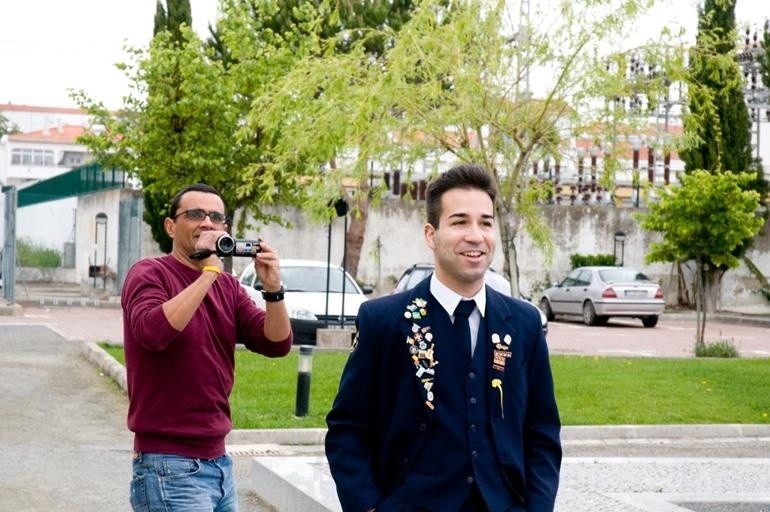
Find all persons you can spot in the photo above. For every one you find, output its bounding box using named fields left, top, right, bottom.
left=325, top=166, right=565, bottom=512
left=118, top=184, right=295, bottom=511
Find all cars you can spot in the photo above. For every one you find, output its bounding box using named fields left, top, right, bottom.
left=536, top=265, right=667, bottom=328
left=391, top=261, right=549, bottom=340
left=238, top=258, right=374, bottom=345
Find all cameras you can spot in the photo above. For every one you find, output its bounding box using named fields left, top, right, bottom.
left=215, top=234, right=261, bottom=258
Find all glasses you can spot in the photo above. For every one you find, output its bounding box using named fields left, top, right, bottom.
left=174, top=209, right=225, bottom=225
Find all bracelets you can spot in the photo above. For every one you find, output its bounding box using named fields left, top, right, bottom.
left=200, top=265, right=224, bottom=276
left=259, top=284, right=286, bottom=302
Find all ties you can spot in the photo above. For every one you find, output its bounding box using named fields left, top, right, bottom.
left=454, top=301, right=478, bottom=358
